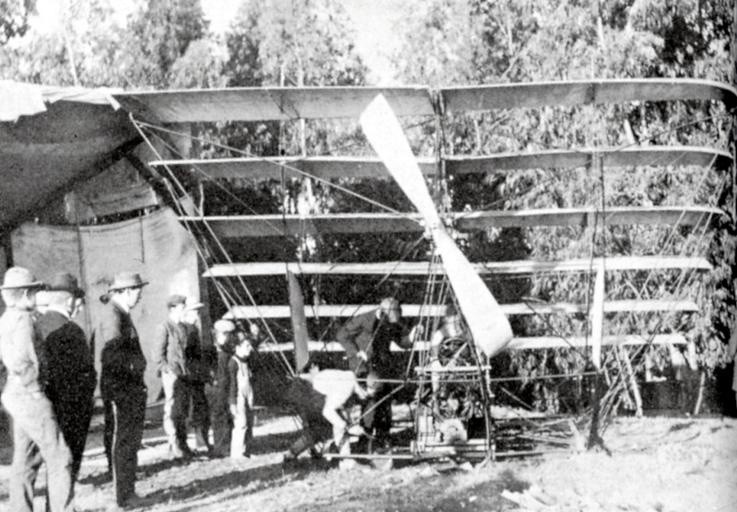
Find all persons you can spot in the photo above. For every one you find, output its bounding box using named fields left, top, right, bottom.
left=154, top=294, right=257, bottom=462
left=283, top=298, right=426, bottom=467
left=0, top=267, right=150, bottom=512
left=726, top=277, right=737, bottom=408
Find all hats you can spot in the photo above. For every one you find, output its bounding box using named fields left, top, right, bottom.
left=167, top=295, right=186, bottom=306
left=214, top=320, right=235, bottom=333
left=0, top=266, right=43, bottom=289
left=46, top=272, right=85, bottom=297
left=109, top=272, right=148, bottom=292
